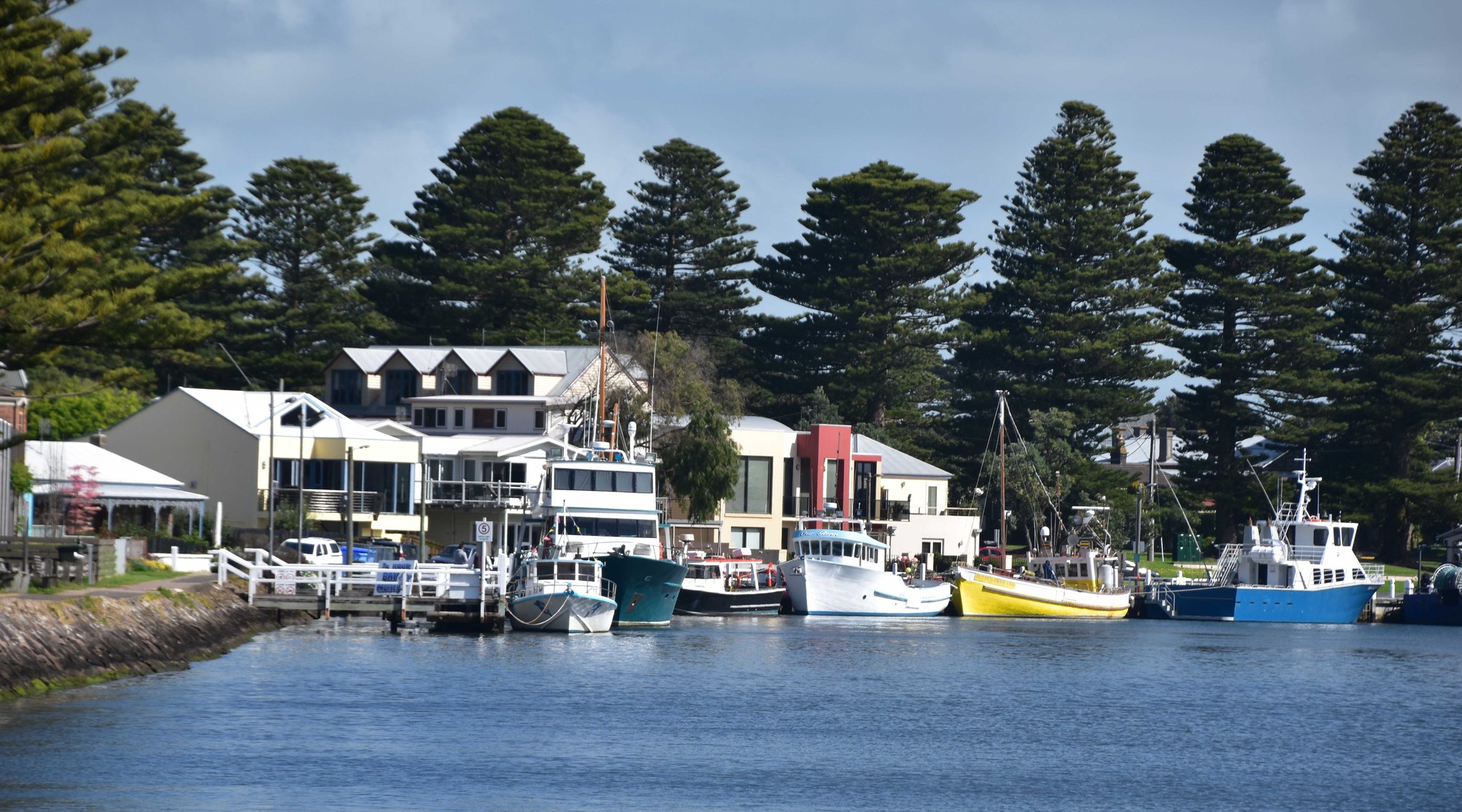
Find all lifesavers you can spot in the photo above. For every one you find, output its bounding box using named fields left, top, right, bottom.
left=739, top=572, right=750, bottom=583
left=725, top=573, right=741, bottom=589
left=1307, top=518, right=1321, bottom=522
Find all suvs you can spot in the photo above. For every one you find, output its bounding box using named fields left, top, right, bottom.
left=373, top=538, right=428, bottom=564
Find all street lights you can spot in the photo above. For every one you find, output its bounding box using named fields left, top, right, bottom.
left=345, top=443, right=369, bottom=588
left=267, top=391, right=298, bottom=591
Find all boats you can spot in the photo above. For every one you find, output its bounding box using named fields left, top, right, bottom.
left=530, top=275, right=690, bottom=627
left=1142, top=447, right=1385, bottom=625
left=673, top=533, right=786, bottom=618
left=507, top=498, right=617, bottom=633
left=776, top=432, right=955, bottom=617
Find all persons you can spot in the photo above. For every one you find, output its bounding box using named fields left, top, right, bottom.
left=453, top=543, right=468, bottom=564
left=531, top=546, right=539, bottom=559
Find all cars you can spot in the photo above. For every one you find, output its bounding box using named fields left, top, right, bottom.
left=979, top=548, right=1003, bottom=562
left=432, top=542, right=477, bottom=567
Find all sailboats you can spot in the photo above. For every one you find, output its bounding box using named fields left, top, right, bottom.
left=951, top=391, right=1134, bottom=620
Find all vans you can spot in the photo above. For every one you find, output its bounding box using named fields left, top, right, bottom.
left=276, top=537, right=343, bottom=573
